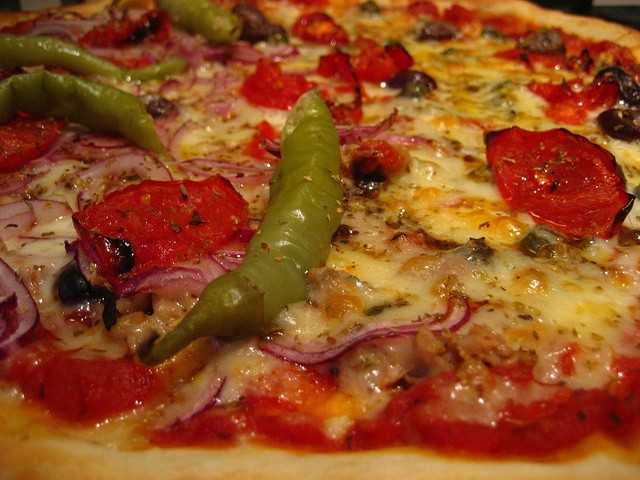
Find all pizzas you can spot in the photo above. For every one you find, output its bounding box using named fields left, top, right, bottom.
left=0, top=0, right=640, bottom=479
